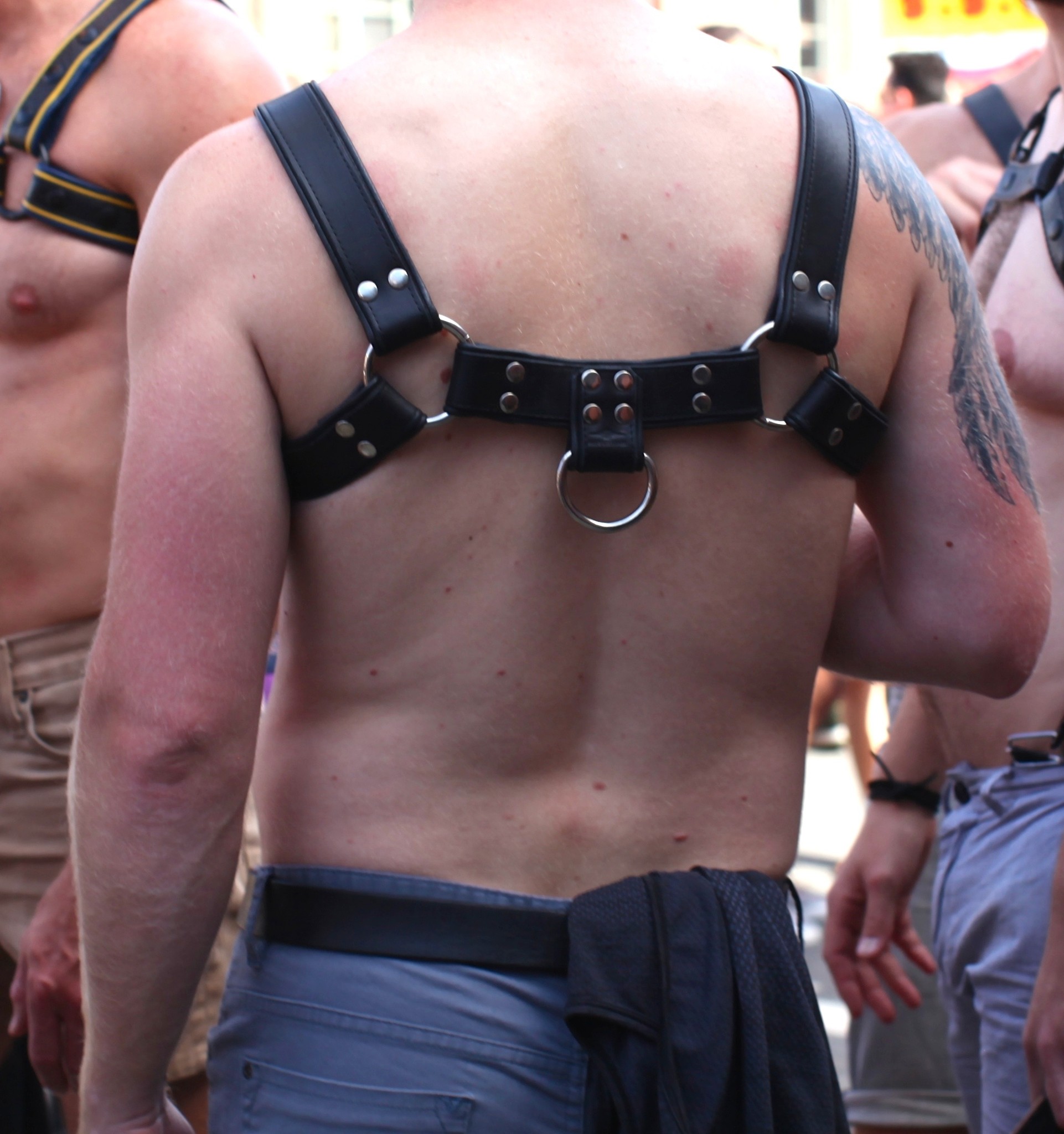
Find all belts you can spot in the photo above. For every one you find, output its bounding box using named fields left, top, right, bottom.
left=238, top=869, right=567, bottom=970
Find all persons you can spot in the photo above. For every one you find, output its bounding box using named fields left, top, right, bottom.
left=0, top=0, right=1064, bottom=1134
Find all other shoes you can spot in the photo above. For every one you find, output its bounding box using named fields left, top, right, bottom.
left=811, top=696, right=851, bottom=747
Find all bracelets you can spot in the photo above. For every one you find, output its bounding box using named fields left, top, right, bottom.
left=863, top=748, right=946, bottom=814
